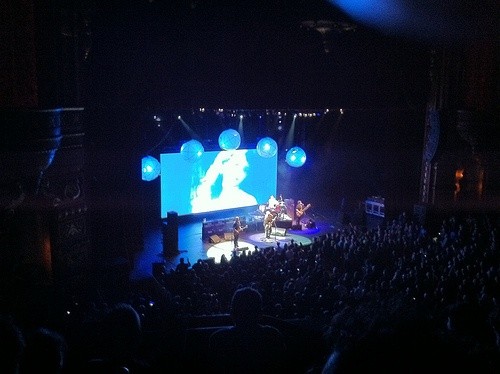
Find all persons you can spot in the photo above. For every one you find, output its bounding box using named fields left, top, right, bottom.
left=191, top=150, right=259, bottom=214
left=0, top=196, right=500, bottom=374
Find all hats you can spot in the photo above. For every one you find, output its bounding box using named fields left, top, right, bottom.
left=231, top=287, right=263, bottom=324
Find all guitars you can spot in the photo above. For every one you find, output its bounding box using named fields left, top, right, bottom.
left=296, top=203, right=311, bottom=217
left=233, top=225, right=248, bottom=236
left=266, top=217, right=276, bottom=230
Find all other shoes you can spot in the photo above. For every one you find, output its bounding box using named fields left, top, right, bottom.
left=266, top=236, right=270, bottom=238
